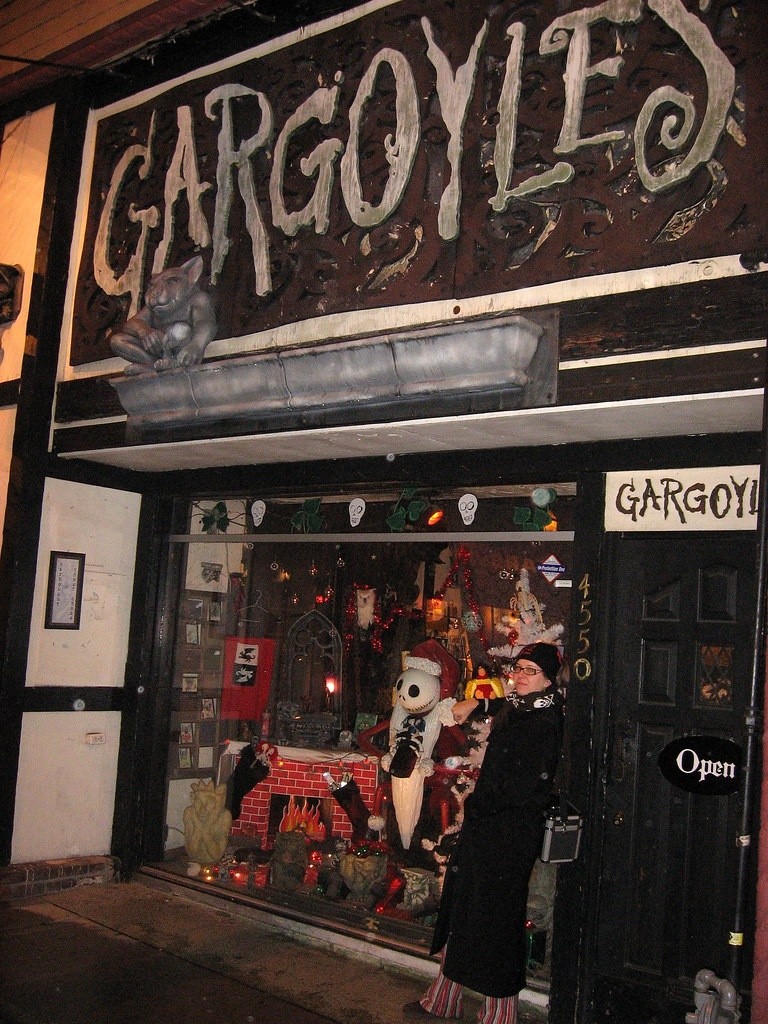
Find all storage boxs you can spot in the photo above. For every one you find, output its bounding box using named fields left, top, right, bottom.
left=536, top=795, right=584, bottom=863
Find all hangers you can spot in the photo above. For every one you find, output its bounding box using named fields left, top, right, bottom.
left=238, top=590, right=285, bottom=624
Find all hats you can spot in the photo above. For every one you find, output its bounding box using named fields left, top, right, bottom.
left=515, top=643, right=561, bottom=684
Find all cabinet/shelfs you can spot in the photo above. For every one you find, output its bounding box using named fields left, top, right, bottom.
left=167, top=589, right=229, bottom=780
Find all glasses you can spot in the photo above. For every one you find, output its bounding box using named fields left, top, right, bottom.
left=510, top=662, right=543, bottom=675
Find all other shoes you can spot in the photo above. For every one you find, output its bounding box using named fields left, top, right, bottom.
left=402, top=1000, right=435, bottom=1020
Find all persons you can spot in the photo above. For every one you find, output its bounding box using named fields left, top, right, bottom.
left=403, top=644, right=567, bottom=1024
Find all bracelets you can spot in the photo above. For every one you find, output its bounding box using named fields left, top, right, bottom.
left=484, top=698, right=489, bottom=713
